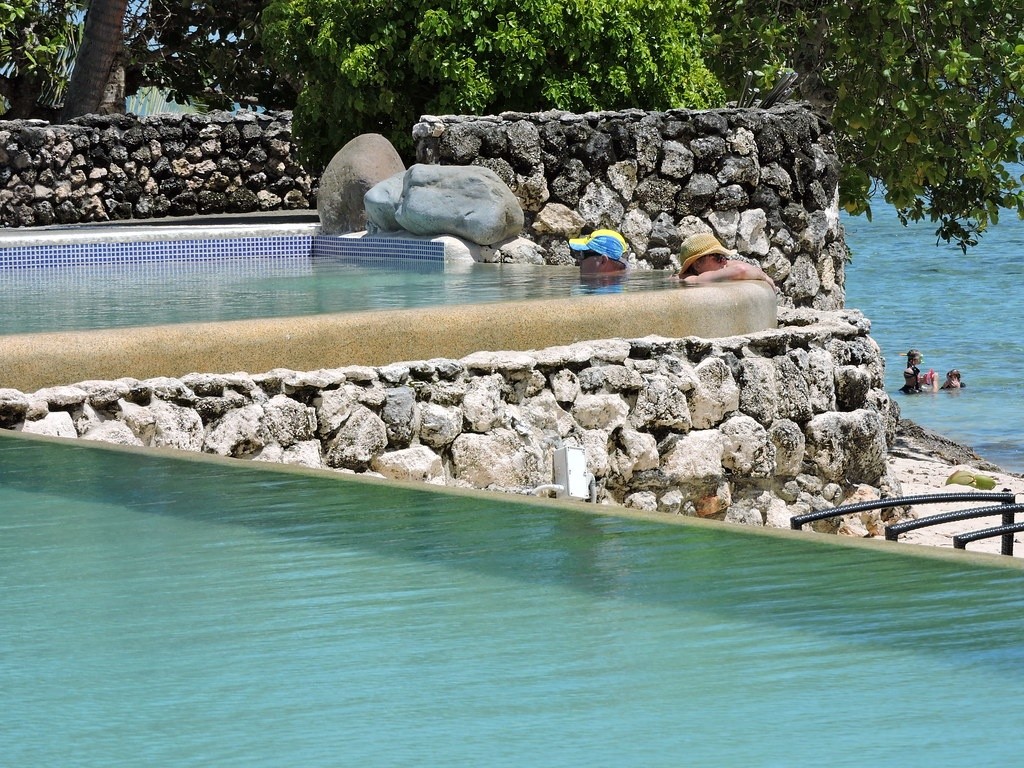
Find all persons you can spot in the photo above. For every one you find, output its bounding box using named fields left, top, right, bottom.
left=569, top=229, right=629, bottom=273
left=674, top=233, right=777, bottom=294
left=899, top=349, right=967, bottom=395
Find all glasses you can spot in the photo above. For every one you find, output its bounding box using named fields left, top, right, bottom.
left=580, top=250, right=602, bottom=260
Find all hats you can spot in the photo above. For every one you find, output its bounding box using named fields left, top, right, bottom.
left=679, top=233, right=731, bottom=279
left=568, top=230, right=626, bottom=261
